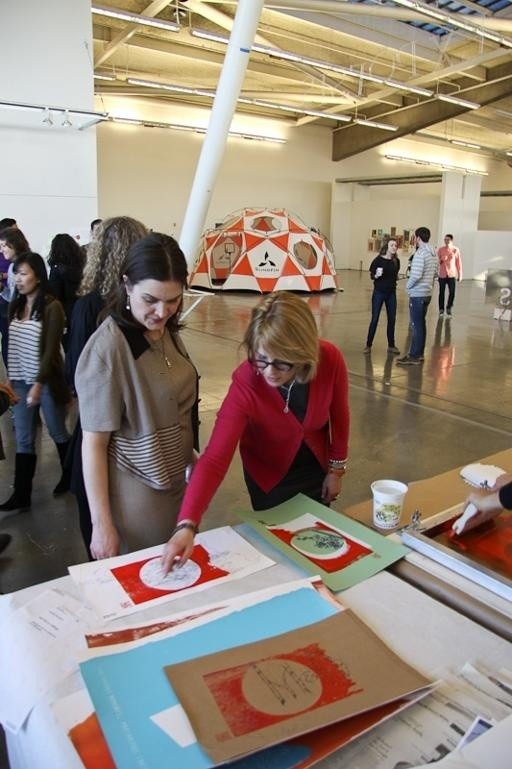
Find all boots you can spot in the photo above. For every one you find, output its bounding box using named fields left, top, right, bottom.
left=1, top=453, right=37, bottom=512
left=53, top=436, right=72, bottom=496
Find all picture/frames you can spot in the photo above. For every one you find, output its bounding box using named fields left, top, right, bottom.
left=399, top=500, right=512, bottom=603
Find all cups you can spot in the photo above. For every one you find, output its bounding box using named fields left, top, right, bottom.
left=376, top=268, right=383, bottom=277
left=370, top=479, right=408, bottom=530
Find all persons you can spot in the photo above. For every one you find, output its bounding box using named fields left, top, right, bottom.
left=466, top=473, right=511, bottom=517
left=361, top=239, right=401, bottom=353
left=0, top=218, right=147, bottom=563
left=407, top=243, right=419, bottom=277
left=75, top=233, right=201, bottom=560
left=436, top=235, right=462, bottom=316
left=160, top=290, right=349, bottom=579
left=396, top=227, right=439, bottom=365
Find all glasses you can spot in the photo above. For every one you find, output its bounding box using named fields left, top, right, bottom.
left=246, top=348, right=294, bottom=371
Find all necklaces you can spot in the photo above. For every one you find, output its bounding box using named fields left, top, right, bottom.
left=279, top=380, right=296, bottom=413
left=147, top=329, right=171, bottom=368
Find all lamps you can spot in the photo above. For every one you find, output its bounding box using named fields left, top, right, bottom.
left=41, top=103, right=75, bottom=131
left=384, top=117, right=512, bottom=177
left=93, top=0, right=512, bottom=144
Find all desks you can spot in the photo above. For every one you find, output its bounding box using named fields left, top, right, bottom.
left=328, top=446, right=512, bottom=642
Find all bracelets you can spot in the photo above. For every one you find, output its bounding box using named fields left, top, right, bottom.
left=170, top=524, right=196, bottom=539
left=329, top=466, right=347, bottom=475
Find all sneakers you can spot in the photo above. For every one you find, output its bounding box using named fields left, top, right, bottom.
left=439, top=310, right=452, bottom=318
left=365, top=345, right=424, bottom=365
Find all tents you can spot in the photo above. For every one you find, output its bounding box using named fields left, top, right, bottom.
left=187, top=207, right=343, bottom=293
